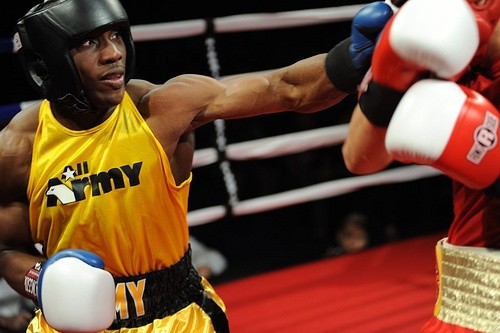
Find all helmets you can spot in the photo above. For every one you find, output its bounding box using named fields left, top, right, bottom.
left=12, top=0, right=134, bottom=118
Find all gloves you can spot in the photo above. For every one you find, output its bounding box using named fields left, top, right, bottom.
left=325, top=0, right=408, bottom=94
left=358, top=0, right=491, bottom=127
left=38, top=248, right=117, bottom=332
left=385, top=79, right=499, bottom=197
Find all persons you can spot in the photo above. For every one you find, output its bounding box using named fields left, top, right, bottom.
left=0, top=276, right=39, bottom=333
left=186, top=233, right=227, bottom=281
left=0, top=0, right=399, bottom=333
left=341, top=0, right=500, bottom=333
left=336, top=213, right=369, bottom=255
